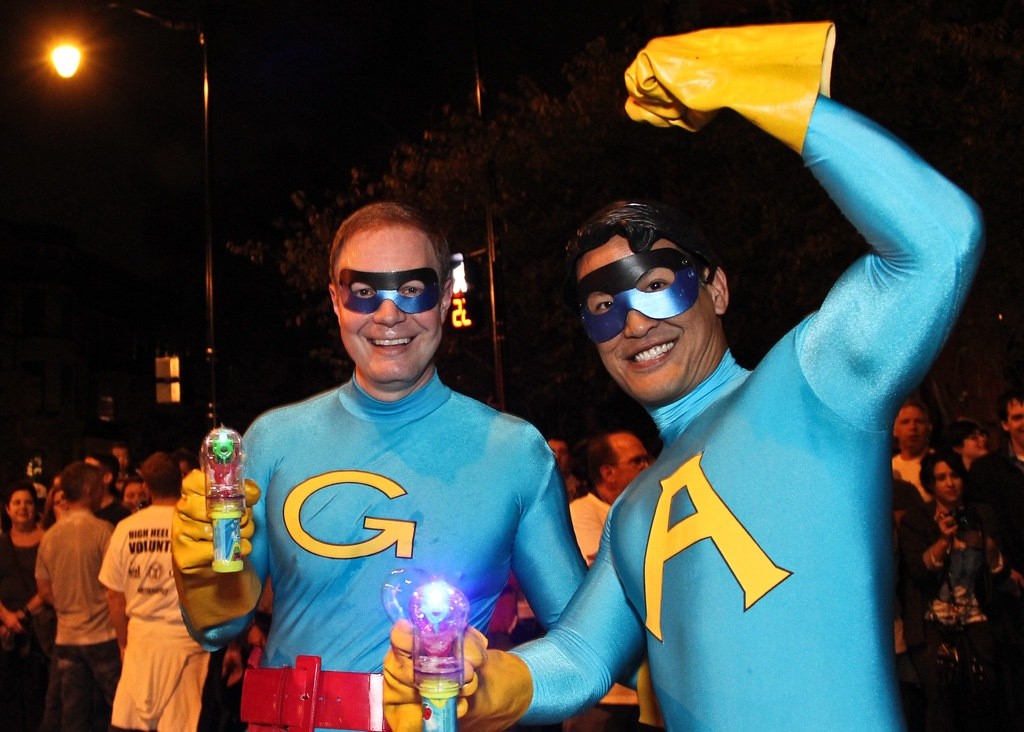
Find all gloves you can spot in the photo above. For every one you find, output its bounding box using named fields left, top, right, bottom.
left=385, top=618, right=534, bottom=732
left=623, top=20, right=836, bottom=154
left=171, top=466, right=262, bottom=631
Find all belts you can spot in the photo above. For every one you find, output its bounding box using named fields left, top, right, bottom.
left=238, top=654, right=394, bottom=732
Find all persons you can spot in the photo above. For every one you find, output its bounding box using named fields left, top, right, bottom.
left=968, top=385, right=1023, bottom=732
left=568, top=431, right=656, bottom=732
left=1, top=433, right=588, bottom=732
left=891, top=477, right=930, bottom=732
left=173, top=204, right=667, bottom=732
left=896, top=446, right=1011, bottom=732
left=889, top=394, right=945, bottom=503
left=944, top=422, right=991, bottom=472
left=380, top=19, right=984, bottom=732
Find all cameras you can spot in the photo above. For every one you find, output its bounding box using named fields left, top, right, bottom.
left=946, top=502, right=983, bottom=533
left=137, top=500, right=149, bottom=511
